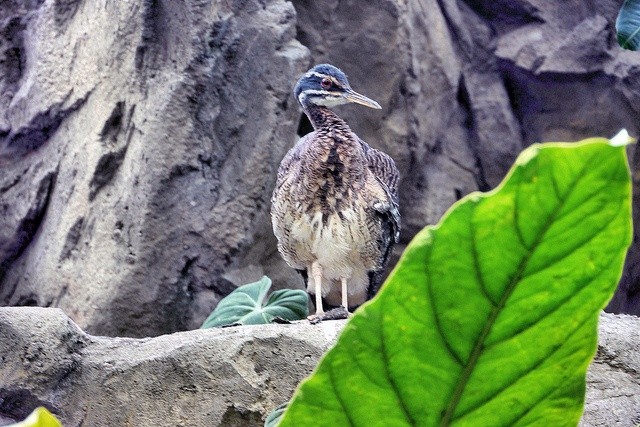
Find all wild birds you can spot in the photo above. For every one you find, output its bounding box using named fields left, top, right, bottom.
left=269, top=63, right=403, bottom=324
